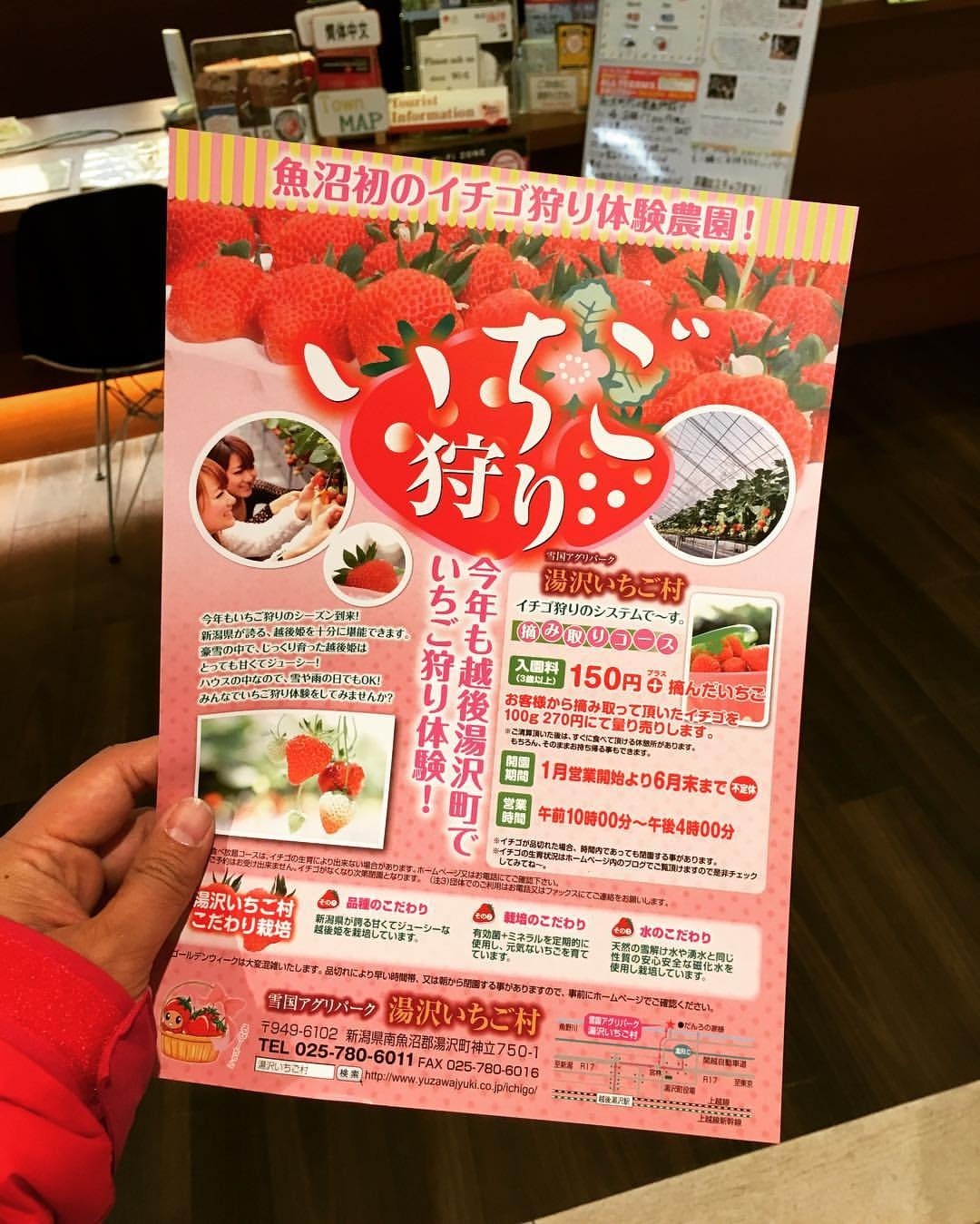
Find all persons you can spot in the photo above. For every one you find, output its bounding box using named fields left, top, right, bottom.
left=195, top=434, right=346, bottom=566
left=0, top=731, right=216, bottom=1224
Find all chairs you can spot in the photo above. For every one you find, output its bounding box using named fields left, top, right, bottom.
left=19, top=182, right=166, bottom=560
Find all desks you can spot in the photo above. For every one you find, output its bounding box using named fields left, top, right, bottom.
left=0, top=96, right=588, bottom=466
